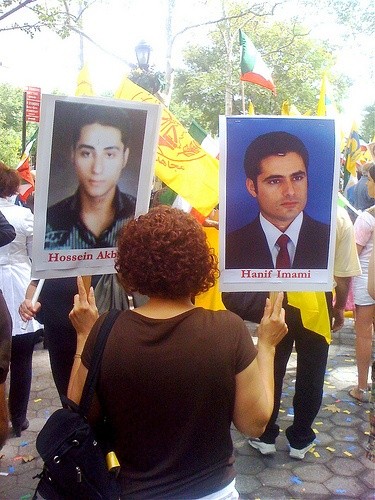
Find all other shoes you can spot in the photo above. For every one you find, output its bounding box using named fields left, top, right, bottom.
left=348, top=387, right=371, bottom=402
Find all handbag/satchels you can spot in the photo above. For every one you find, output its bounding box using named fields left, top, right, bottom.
left=32, top=395, right=124, bottom=500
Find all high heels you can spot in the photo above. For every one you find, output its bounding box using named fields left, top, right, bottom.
left=14, top=418, right=30, bottom=437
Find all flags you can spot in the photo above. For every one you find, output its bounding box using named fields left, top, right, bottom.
left=239, top=28, right=277, bottom=93
left=161, top=121, right=220, bottom=224
left=15, top=127, right=38, bottom=201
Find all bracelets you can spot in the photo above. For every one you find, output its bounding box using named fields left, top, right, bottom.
left=74, top=353, right=82, bottom=358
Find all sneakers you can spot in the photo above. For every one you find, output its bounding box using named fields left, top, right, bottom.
left=289, top=441, right=314, bottom=459
left=248, top=436, right=277, bottom=456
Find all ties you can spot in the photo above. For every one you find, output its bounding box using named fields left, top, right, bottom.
left=275, top=234, right=291, bottom=269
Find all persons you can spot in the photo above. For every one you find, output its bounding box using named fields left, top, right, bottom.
left=44, top=106, right=136, bottom=249
left=0, top=162, right=375, bottom=462
left=67, top=206, right=289, bottom=500
left=225, top=131, right=330, bottom=269
